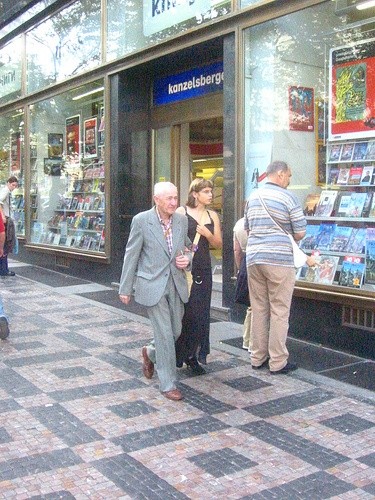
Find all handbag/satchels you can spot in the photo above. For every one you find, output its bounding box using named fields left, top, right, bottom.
left=291, top=236, right=310, bottom=270
left=185, top=269, right=192, bottom=298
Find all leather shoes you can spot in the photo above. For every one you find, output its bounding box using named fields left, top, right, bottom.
left=252, top=356, right=269, bottom=369
left=185, top=360, right=205, bottom=374
left=143, top=347, right=154, bottom=378
left=176, top=357, right=183, bottom=367
left=161, top=389, right=183, bottom=400
left=270, top=361, right=297, bottom=373
left=6, top=271, right=15, bottom=276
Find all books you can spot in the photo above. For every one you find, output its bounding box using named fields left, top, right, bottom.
left=11, top=155, right=105, bottom=253
left=296, top=140, right=375, bottom=289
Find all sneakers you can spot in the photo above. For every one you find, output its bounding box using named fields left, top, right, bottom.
left=0, top=316, right=9, bottom=339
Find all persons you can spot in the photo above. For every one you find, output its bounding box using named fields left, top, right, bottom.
left=233, top=201, right=321, bottom=353
left=244, top=161, right=307, bottom=374
left=85, top=129, right=95, bottom=155
left=176, top=178, right=222, bottom=375
left=0, top=176, right=18, bottom=275
left=0, top=209, right=10, bottom=340
left=67, top=132, right=76, bottom=155
left=119, top=182, right=192, bottom=401
